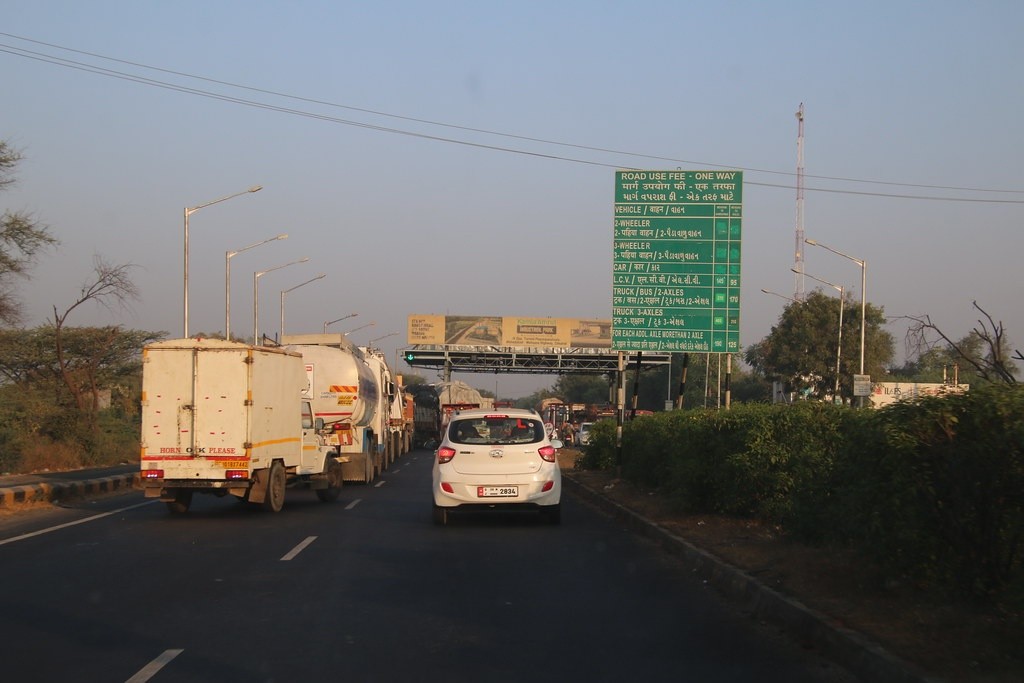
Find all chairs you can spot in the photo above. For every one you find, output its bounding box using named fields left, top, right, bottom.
left=458, top=427, right=482, bottom=442
left=508, top=422, right=530, bottom=437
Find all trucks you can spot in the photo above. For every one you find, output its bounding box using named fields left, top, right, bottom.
left=141, top=337, right=344, bottom=516
left=435, top=402, right=481, bottom=441
left=568, top=403, right=585, bottom=411
left=540, top=405, right=570, bottom=422
left=262, top=333, right=395, bottom=486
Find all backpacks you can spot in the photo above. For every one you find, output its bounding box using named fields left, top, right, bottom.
left=566, top=423, right=572, bottom=434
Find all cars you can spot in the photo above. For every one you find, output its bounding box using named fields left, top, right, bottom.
left=424, top=408, right=562, bottom=526
left=574, top=422, right=596, bottom=445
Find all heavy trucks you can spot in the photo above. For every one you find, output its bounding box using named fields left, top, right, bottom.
left=385, top=368, right=441, bottom=465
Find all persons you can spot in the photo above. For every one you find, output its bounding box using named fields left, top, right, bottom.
left=561, top=420, right=577, bottom=443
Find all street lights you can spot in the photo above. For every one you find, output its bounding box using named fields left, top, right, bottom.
left=369, top=331, right=400, bottom=348
left=792, top=268, right=844, bottom=405
left=805, top=239, right=866, bottom=408
left=183, top=185, right=266, bottom=337
left=281, top=273, right=327, bottom=335
left=225, top=232, right=289, bottom=341
left=254, top=259, right=310, bottom=346
left=343, top=322, right=376, bottom=336
left=761, top=288, right=802, bottom=402
left=323, top=314, right=358, bottom=334
